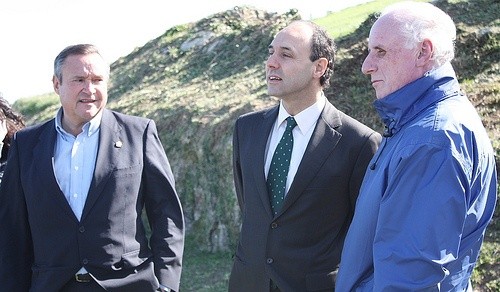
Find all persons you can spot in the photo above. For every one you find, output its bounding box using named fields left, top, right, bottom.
left=0, top=45, right=185, bottom=291
left=227, top=20, right=383, bottom=292
left=1, top=96, right=24, bottom=186
left=334, top=3, right=497, bottom=292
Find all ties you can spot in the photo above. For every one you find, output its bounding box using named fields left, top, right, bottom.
left=265, top=116, right=297, bottom=219
